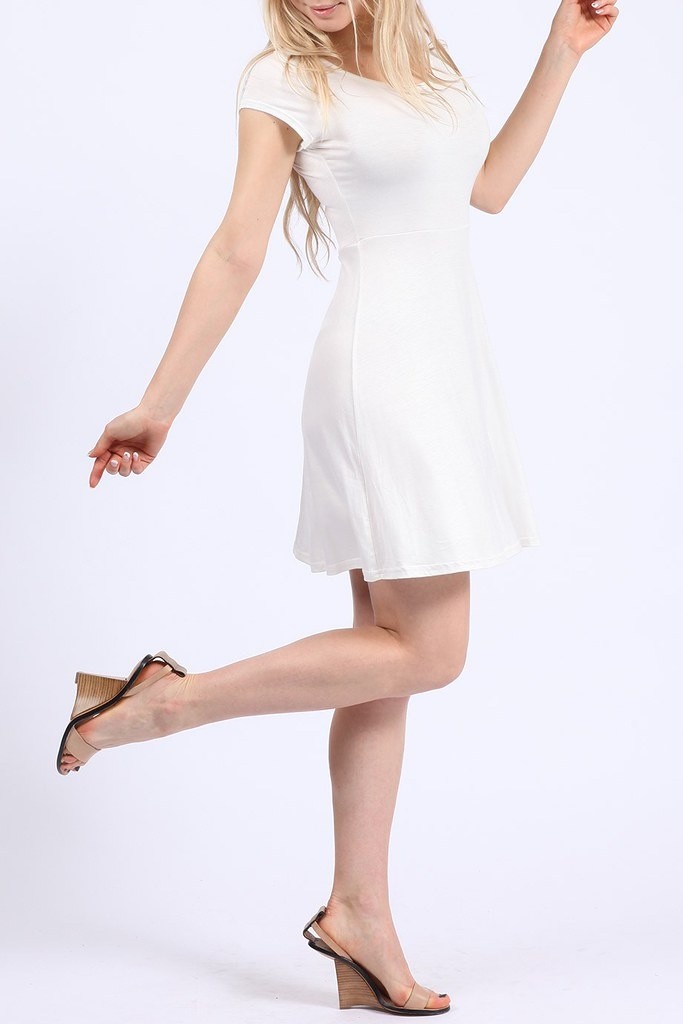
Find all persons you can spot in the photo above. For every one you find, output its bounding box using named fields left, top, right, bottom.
left=57, top=0, right=618, bottom=1016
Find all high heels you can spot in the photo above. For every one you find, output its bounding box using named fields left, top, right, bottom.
left=55, top=650, right=186, bottom=777
left=304, top=908, right=450, bottom=1016
